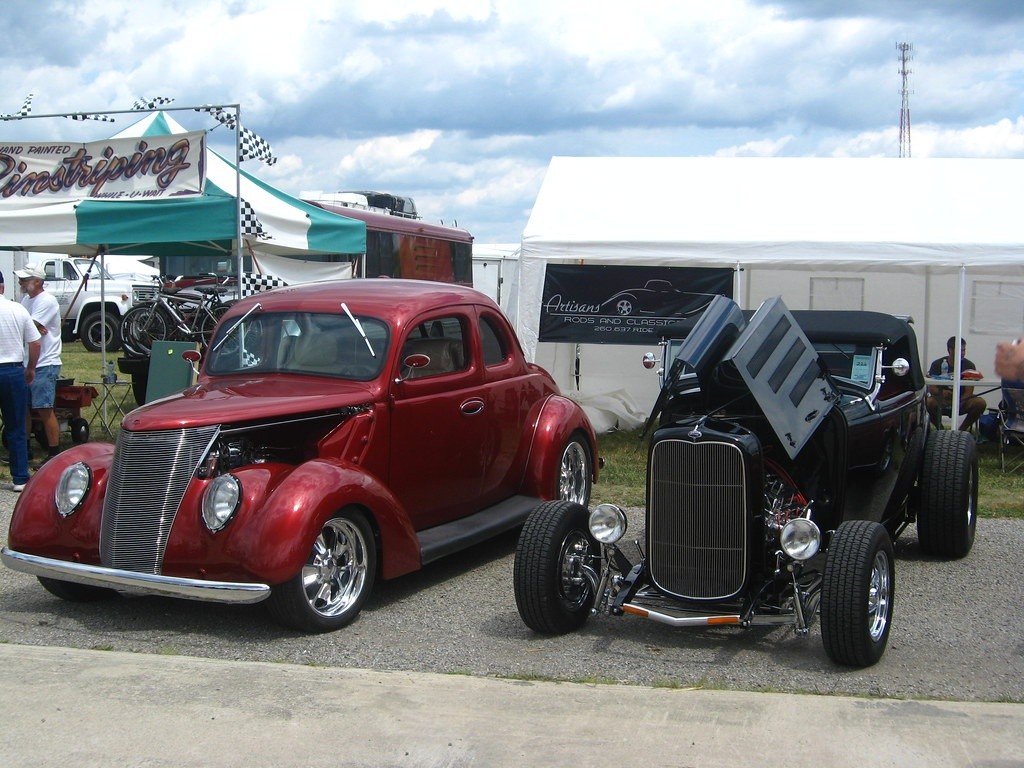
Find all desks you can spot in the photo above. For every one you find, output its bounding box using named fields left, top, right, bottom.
left=923, top=378, right=1002, bottom=445
left=79, top=381, right=136, bottom=439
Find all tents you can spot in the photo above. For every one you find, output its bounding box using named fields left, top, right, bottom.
left=0, top=109, right=368, bottom=426
left=472, top=154, right=1024, bottom=434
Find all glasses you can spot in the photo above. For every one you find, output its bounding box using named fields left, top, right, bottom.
left=19, top=277, right=35, bottom=281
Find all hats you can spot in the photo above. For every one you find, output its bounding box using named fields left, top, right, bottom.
left=13, top=263, right=46, bottom=280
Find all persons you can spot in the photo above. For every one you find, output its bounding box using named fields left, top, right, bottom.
left=994, top=338, right=1024, bottom=382
left=0, top=272, right=41, bottom=492
left=12, top=263, right=62, bottom=472
left=925, top=335, right=987, bottom=431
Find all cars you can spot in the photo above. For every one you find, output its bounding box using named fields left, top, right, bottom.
left=513, top=294, right=982, bottom=664
left=1, top=275, right=603, bottom=638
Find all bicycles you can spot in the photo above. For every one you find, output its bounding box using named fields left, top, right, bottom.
left=118, top=269, right=263, bottom=362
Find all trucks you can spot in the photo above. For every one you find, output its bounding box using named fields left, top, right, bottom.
left=1, top=250, right=160, bottom=352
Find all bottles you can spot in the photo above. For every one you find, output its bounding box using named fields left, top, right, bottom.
left=108, top=360, right=114, bottom=384
left=941, top=359, right=948, bottom=379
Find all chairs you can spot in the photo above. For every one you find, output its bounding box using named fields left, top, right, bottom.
left=919, top=392, right=978, bottom=443
left=1000, top=387, right=1024, bottom=472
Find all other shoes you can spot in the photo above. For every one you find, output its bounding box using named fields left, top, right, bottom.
left=33, top=455, right=54, bottom=471
left=1, top=449, right=33, bottom=463
left=13, top=483, right=25, bottom=492
